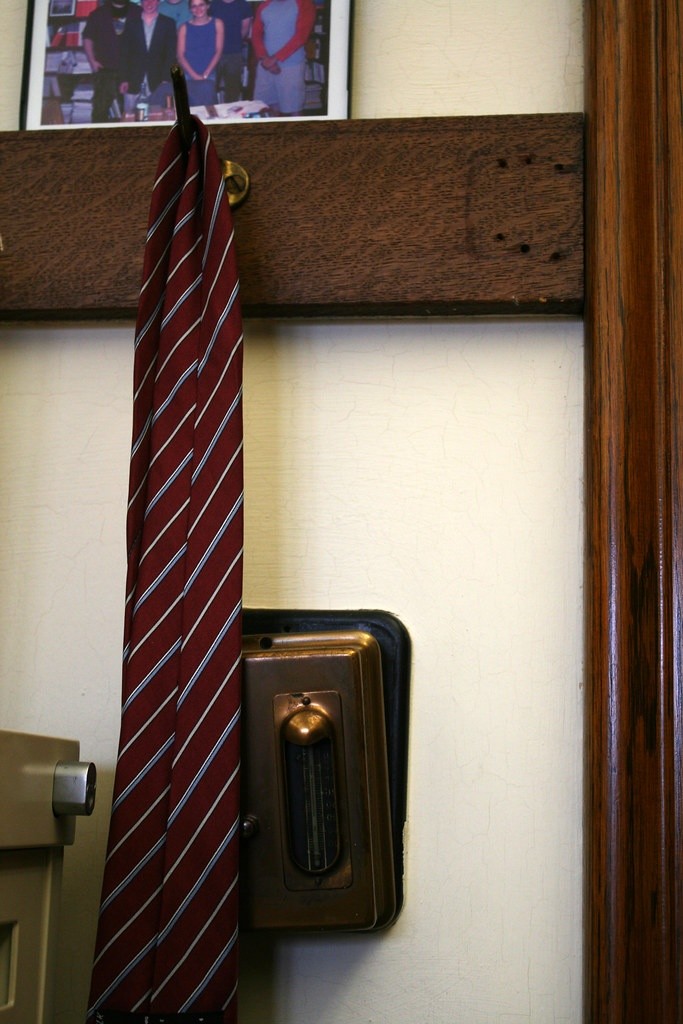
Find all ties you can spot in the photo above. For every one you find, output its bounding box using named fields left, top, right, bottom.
left=85, top=115, right=243, bottom=1024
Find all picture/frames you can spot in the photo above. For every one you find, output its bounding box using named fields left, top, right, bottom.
left=18, top=0, right=354, bottom=130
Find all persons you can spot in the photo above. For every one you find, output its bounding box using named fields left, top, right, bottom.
left=207, top=1, right=317, bottom=117
left=176, top=0, right=224, bottom=106
left=82, top=0, right=177, bottom=123
